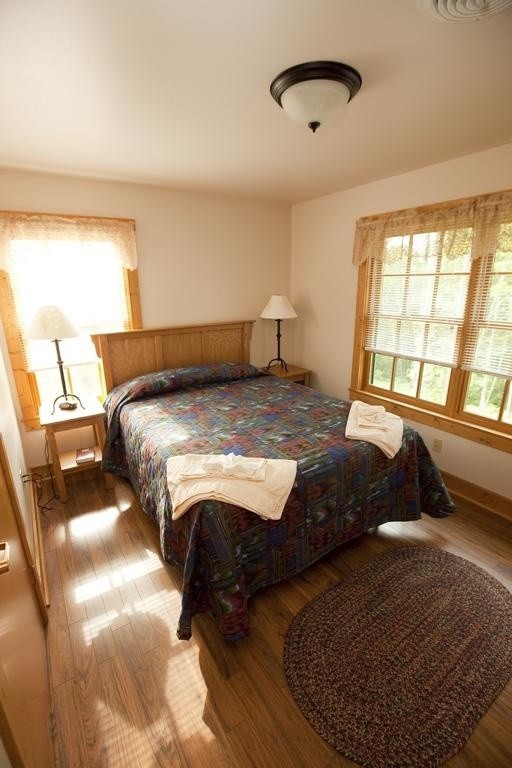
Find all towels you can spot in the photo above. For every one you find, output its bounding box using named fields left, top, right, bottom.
left=167, top=455, right=298, bottom=521
left=344, top=400, right=405, bottom=460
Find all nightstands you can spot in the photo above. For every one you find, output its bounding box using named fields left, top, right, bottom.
left=262, top=366, right=313, bottom=387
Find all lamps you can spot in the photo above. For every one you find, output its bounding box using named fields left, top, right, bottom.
left=260, top=295, right=298, bottom=373
left=27, top=305, right=86, bottom=414
left=270, top=62, right=362, bottom=134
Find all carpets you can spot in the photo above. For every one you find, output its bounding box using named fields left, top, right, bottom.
left=283, top=546, right=510, bottom=768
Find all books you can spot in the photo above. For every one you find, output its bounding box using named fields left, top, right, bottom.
left=74, top=445, right=96, bottom=463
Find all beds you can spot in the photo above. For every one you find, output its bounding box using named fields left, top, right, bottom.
left=90, top=320, right=417, bottom=642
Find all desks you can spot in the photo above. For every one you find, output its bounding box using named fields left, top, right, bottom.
left=39, top=398, right=116, bottom=504
